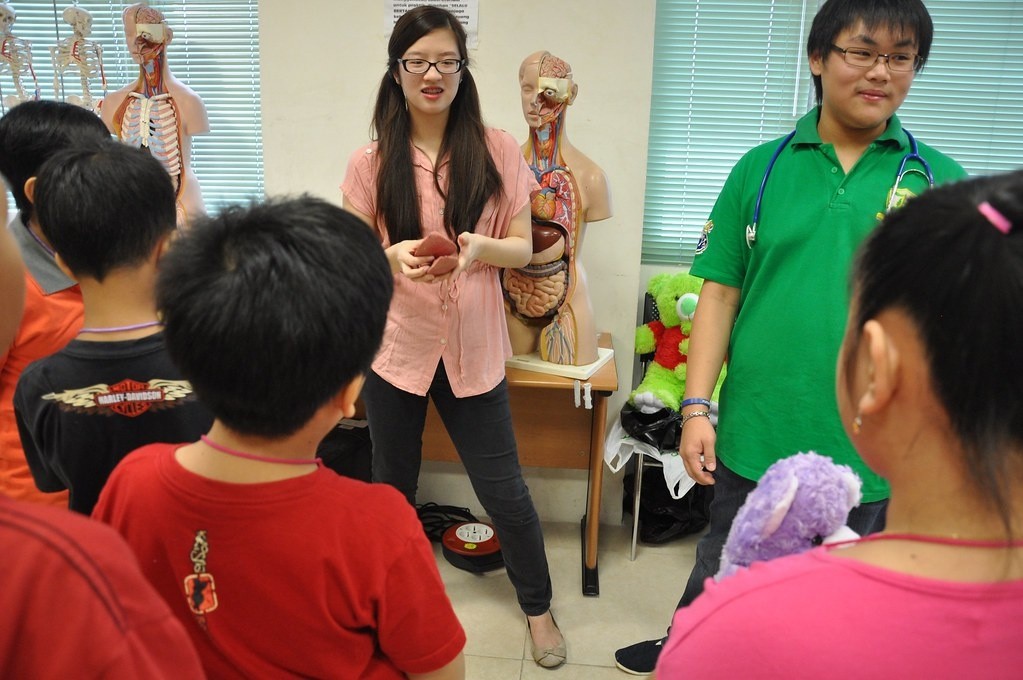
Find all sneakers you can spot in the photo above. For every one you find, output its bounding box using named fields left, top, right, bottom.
left=615, top=637, right=667, bottom=676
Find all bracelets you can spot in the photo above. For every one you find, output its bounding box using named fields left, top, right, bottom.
left=680, top=398, right=711, bottom=409
left=681, top=411, right=711, bottom=427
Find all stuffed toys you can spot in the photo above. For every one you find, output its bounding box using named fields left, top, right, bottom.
left=712, top=451, right=864, bottom=580
left=628, top=272, right=729, bottom=426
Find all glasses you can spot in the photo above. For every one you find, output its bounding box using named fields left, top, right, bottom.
left=398, top=58, right=465, bottom=76
left=832, top=44, right=923, bottom=73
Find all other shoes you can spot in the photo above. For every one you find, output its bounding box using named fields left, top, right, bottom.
left=525, top=608, right=567, bottom=668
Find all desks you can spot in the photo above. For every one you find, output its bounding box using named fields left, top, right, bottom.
left=354, top=333, right=617, bottom=596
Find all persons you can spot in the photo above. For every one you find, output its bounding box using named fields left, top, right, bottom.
left=614, top=0, right=968, bottom=676
left=656, top=169, right=1023, bottom=680
left=501, top=50, right=613, bottom=367
left=0, top=100, right=469, bottom=680
left=98, top=5, right=212, bottom=229
left=342, top=7, right=569, bottom=672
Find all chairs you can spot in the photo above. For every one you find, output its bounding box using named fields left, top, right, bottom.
left=630, top=293, right=723, bottom=562
